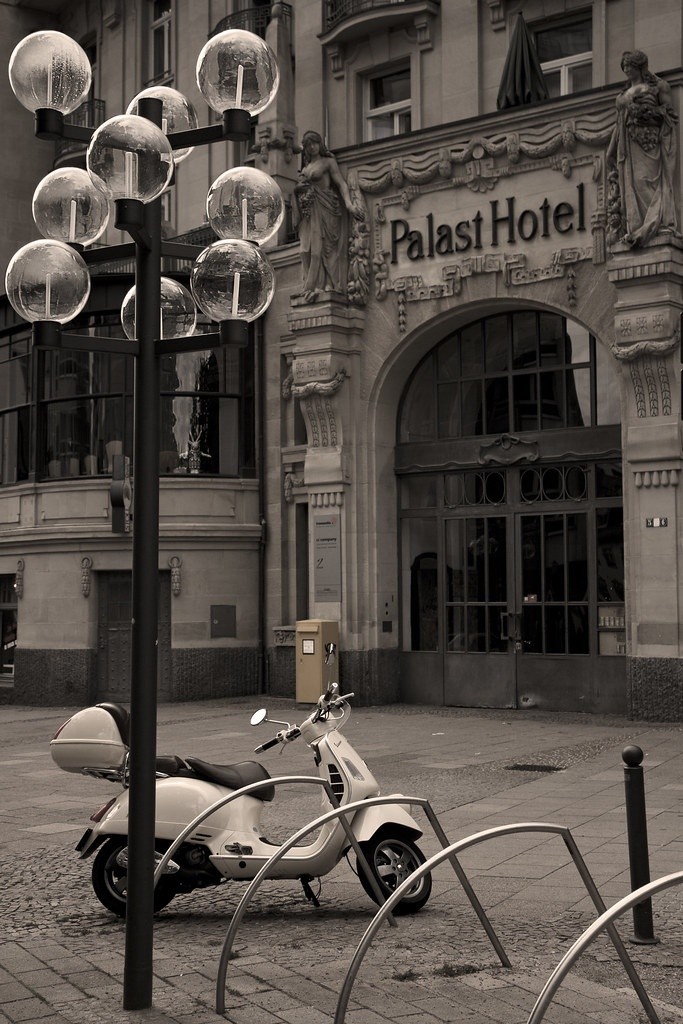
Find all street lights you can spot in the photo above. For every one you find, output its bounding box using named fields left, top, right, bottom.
left=4, top=28, right=285, bottom=1010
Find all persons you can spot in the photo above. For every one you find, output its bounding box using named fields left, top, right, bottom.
left=292, top=131, right=364, bottom=297
left=606, top=49, right=679, bottom=244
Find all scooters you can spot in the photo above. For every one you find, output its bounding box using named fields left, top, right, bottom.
left=48, top=641, right=434, bottom=920
